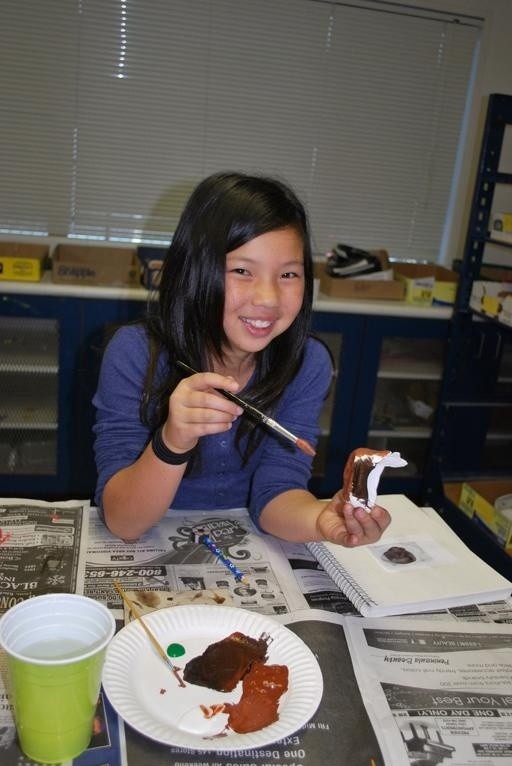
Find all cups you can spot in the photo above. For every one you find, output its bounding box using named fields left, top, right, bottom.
left=0, top=592, right=118, bottom=766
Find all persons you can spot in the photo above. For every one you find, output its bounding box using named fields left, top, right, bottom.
left=92, top=169, right=393, bottom=548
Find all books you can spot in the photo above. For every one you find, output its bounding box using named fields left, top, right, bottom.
left=303, top=492, right=512, bottom=617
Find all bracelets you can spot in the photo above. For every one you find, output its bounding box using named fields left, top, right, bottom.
left=153, top=423, right=198, bottom=465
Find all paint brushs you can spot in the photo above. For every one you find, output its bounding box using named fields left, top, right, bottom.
left=113, top=579, right=185, bottom=688
left=177, top=360, right=316, bottom=457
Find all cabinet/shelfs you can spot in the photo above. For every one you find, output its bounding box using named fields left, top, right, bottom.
left=2, top=276, right=453, bottom=507
left=410, top=91, right=511, bottom=507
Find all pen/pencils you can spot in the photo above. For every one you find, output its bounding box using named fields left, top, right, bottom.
left=203, top=536, right=248, bottom=585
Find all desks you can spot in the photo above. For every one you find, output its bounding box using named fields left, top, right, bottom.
left=0, top=495, right=510, bottom=766
